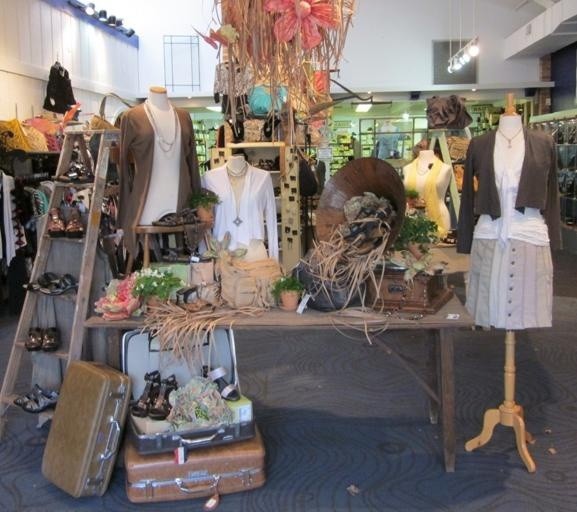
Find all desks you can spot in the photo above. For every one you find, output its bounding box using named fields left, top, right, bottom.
left=82, top=288, right=476, bottom=472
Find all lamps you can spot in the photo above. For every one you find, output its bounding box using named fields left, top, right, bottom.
left=83, top=2, right=135, bottom=39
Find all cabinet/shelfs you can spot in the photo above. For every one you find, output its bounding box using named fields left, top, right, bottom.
left=530, top=116, right=576, bottom=235
left=123, top=218, right=214, bottom=278
left=208, top=143, right=302, bottom=274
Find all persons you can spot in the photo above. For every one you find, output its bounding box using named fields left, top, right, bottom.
left=372, top=118, right=402, bottom=158
left=402, top=147, right=453, bottom=242
left=113, top=85, right=203, bottom=257
left=199, top=151, right=282, bottom=262
left=451, top=111, right=566, bottom=330
left=44, top=67, right=78, bottom=113
left=48, top=61, right=70, bottom=79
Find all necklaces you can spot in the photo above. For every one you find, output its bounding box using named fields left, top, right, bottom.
left=414, top=161, right=433, bottom=176
left=225, top=162, right=249, bottom=177
left=496, top=126, right=524, bottom=149
left=146, top=102, right=179, bottom=153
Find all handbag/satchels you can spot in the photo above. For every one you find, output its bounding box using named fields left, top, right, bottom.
left=191, top=262, right=214, bottom=285
left=426, top=95, right=472, bottom=129
left=292, top=248, right=367, bottom=311
left=221, top=258, right=287, bottom=310
left=454, top=167, right=478, bottom=193
left=446, top=136, right=470, bottom=161
left=152, top=208, right=200, bottom=225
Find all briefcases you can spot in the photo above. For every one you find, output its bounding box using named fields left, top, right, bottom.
left=40, top=322, right=267, bottom=505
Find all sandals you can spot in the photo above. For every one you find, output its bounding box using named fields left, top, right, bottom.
left=14, top=383, right=60, bottom=414
left=48, top=206, right=84, bottom=238
left=26, top=327, right=42, bottom=350
left=51, top=161, right=94, bottom=183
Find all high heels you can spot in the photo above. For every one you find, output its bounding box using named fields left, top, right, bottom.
left=28, top=273, right=79, bottom=296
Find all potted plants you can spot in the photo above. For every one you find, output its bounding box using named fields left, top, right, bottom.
left=271, top=276, right=304, bottom=311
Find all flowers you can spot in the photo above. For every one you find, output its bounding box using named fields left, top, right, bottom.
left=131, top=268, right=180, bottom=301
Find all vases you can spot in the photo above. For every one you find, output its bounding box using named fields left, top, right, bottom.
left=145, top=294, right=167, bottom=312
